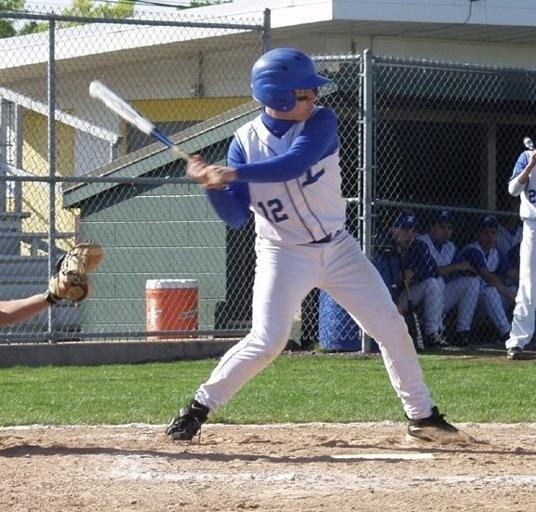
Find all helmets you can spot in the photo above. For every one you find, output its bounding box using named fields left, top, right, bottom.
left=249, top=48, right=333, bottom=114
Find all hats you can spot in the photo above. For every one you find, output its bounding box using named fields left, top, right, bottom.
left=479, top=214, right=499, bottom=229
left=431, top=208, right=456, bottom=225
left=392, top=211, right=421, bottom=229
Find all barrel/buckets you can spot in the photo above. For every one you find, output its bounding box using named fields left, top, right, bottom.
left=146, top=278, right=199, bottom=338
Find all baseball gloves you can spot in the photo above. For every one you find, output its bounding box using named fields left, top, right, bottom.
left=47, top=243, right=104, bottom=302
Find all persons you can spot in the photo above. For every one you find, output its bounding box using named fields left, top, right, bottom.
left=449, top=214, right=516, bottom=341
left=163, top=45, right=465, bottom=443
left=498, top=211, right=521, bottom=284
left=0, top=242, right=105, bottom=326
left=375, top=209, right=447, bottom=347
left=502, top=147, right=536, bottom=358
left=420, top=209, right=479, bottom=343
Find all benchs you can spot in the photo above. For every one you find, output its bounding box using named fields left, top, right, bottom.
left=0, top=207, right=93, bottom=291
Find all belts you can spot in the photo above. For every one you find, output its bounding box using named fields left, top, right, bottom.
left=308, top=229, right=344, bottom=244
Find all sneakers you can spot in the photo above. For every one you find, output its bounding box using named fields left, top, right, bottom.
left=507, top=347, right=525, bottom=359
left=164, top=399, right=210, bottom=442
left=404, top=405, right=478, bottom=446
left=423, top=327, right=516, bottom=349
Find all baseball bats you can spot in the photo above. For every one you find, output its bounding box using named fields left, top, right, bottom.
left=398, top=257, right=424, bottom=352
left=88, top=80, right=231, bottom=191
